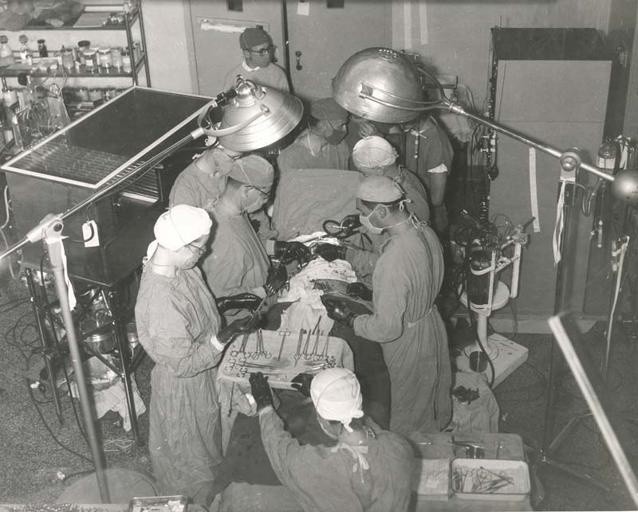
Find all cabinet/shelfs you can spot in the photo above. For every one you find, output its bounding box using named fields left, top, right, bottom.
left=0, top=1, right=151, bottom=156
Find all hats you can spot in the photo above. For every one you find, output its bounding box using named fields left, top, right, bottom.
left=311, top=97, right=350, bottom=121
left=154, top=205, right=213, bottom=249
left=353, top=135, right=398, bottom=167
left=310, top=367, right=363, bottom=421
left=355, top=176, right=403, bottom=203
left=239, top=27, right=269, bottom=50
left=229, top=155, right=274, bottom=187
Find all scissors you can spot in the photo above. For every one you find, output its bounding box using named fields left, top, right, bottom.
left=230, top=325, right=336, bottom=377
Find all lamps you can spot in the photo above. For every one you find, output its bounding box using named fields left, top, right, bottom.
left=331, top=47, right=616, bottom=493
left=0, top=76, right=304, bottom=506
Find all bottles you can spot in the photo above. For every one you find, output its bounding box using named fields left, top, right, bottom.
left=0, top=34, right=141, bottom=110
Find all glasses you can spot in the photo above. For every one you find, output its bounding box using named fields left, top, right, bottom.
left=189, top=244, right=209, bottom=257
left=217, top=148, right=239, bottom=161
left=253, top=187, right=274, bottom=199
left=250, top=46, right=275, bottom=56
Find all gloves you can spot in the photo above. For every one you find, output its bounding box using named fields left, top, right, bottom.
left=322, top=297, right=356, bottom=328
left=341, top=214, right=362, bottom=234
left=249, top=372, right=273, bottom=411
left=292, top=373, right=312, bottom=397
left=217, top=316, right=256, bottom=345
left=347, top=282, right=372, bottom=301
left=264, top=265, right=287, bottom=295
left=315, top=243, right=347, bottom=261
left=216, top=293, right=267, bottom=315
left=274, top=240, right=311, bottom=265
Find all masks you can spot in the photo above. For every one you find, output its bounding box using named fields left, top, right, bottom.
left=359, top=204, right=391, bottom=234
left=250, top=53, right=273, bottom=66
left=172, top=247, right=199, bottom=270
left=317, top=415, right=338, bottom=440
left=242, top=190, right=268, bottom=213
left=317, top=121, right=347, bottom=145
left=218, top=156, right=233, bottom=173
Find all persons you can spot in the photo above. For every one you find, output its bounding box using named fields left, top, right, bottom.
left=129, top=205, right=269, bottom=511
left=320, top=175, right=452, bottom=445
left=168, top=96, right=274, bottom=329
left=351, top=97, right=453, bottom=244
left=218, top=27, right=292, bottom=97
left=250, top=366, right=415, bottom=510
